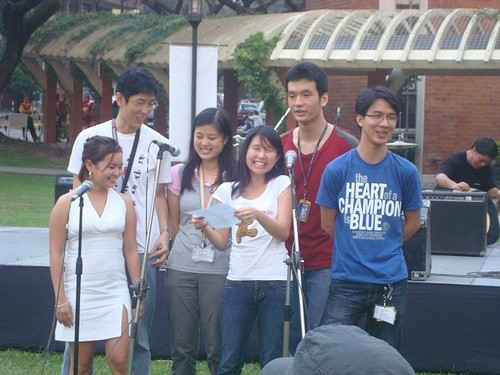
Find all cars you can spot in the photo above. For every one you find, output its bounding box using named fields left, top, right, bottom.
left=257, top=100, right=267, bottom=121
left=236, top=103, right=257, bottom=126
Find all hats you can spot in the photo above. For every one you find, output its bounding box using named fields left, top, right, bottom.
left=260, top=324, right=416, bottom=375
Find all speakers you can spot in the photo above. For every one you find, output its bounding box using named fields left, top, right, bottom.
left=54, top=175, right=73, bottom=205
left=401, top=190, right=489, bottom=280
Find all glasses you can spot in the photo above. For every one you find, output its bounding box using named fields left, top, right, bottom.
left=362, top=114, right=399, bottom=124
left=122, top=95, right=159, bottom=111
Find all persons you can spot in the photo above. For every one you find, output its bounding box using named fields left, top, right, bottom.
left=435, top=137, right=500, bottom=245
left=164, top=107, right=236, bottom=375
left=47, top=134, right=145, bottom=375
left=192, top=124, right=293, bottom=375
left=316, top=85, right=423, bottom=352
left=19, top=97, right=40, bottom=143
left=61, top=64, right=172, bottom=375
left=261, top=324, right=416, bottom=375
left=56, top=94, right=97, bottom=143
left=279, top=61, right=368, bottom=338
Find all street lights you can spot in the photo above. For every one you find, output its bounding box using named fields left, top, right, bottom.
left=187, top=0, right=205, bottom=157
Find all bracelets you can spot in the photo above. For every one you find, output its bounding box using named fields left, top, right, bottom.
left=161, top=228, right=172, bottom=240
left=55, top=301, right=68, bottom=308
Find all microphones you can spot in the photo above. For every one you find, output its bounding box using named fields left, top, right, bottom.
left=153, top=140, right=181, bottom=157
left=70, top=180, right=93, bottom=202
left=285, top=150, right=298, bottom=170
